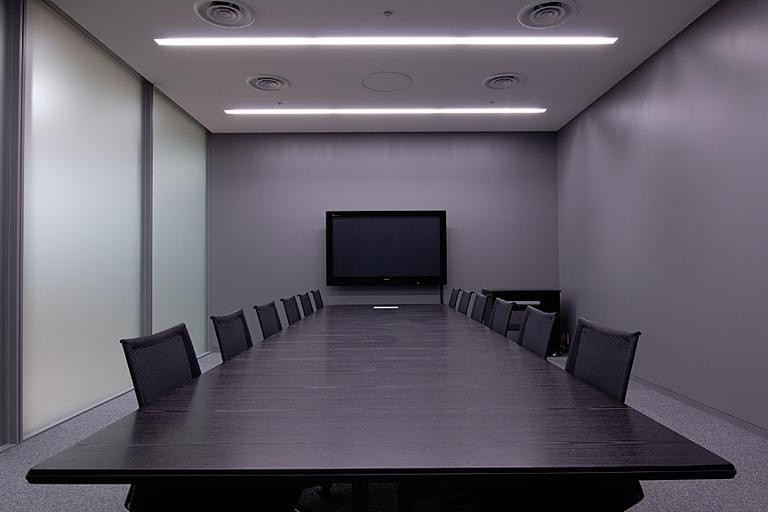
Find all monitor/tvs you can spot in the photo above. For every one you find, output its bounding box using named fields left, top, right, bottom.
left=326, top=210, right=448, bottom=290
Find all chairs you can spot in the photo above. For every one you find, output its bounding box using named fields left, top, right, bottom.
left=119, top=320, right=305, bottom=509
left=488, top=298, right=515, bottom=338
left=470, top=292, right=489, bottom=323
left=310, top=288, right=324, bottom=311
left=208, top=307, right=253, bottom=363
left=515, top=305, right=558, bottom=360
left=448, top=288, right=462, bottom=308
left=459, top=315, right=646, bottom=510
left=280, top=295, right=302, bottom=326
left=296, top=291, right=314, bottom=317
left=458, top=289, right=475, bottom=315
left=253, top=299, right=284, bottom=340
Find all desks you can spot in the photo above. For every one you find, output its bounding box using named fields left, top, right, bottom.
left=25, top=304, right=738, bottom=509
left=479, top=288, right=562, bottom=357
